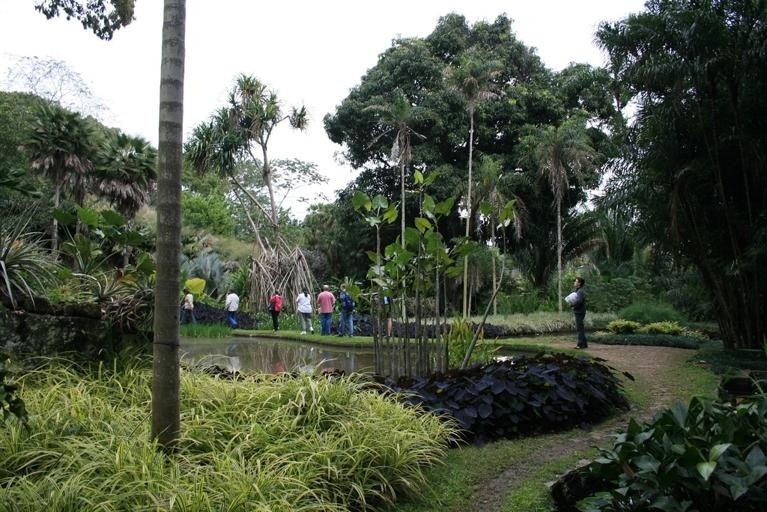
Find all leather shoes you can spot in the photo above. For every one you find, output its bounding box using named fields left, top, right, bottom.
left=575, top=346, right=581, bottom=349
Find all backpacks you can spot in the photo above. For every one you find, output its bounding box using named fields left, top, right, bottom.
left=343, top=295, right=355, bottom=310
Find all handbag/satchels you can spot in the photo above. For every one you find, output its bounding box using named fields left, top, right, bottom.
left=268, top=303, right=275, bottom=310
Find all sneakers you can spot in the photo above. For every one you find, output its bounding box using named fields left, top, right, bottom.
left=301, top=327, right=314, bottom=335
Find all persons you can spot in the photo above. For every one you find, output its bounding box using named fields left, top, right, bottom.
left=179, top=286, right=197, bottom=326
left=225, top=289, right=239, bottom=329
left=376, top=296, right=393, bottom=339
left=335, top=283, right=353, bottom=338
left=316, top=285, right=335, bottom=337
left=569, top=277, right=588, bottom=349
left=295, top=287, right=315, bottom=336
left=269, top=290, right=283, bottom=333
